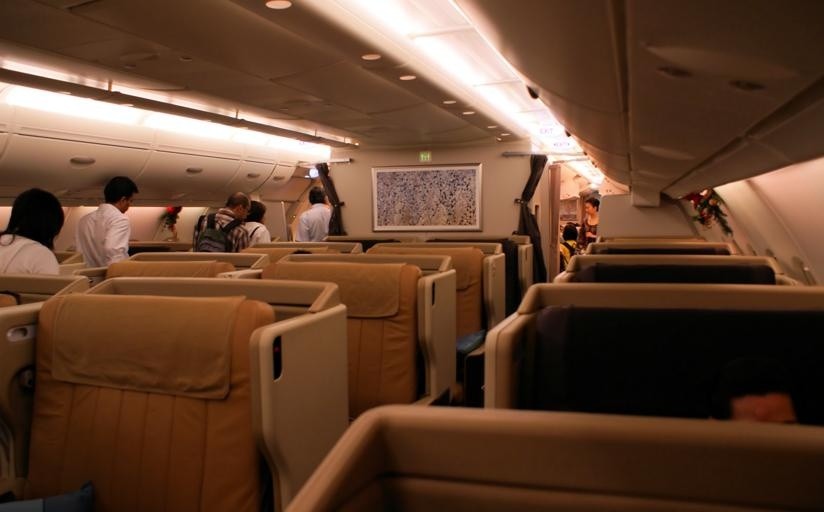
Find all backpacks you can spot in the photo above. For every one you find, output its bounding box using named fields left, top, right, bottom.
left=198, top=213, right=241, bottom=252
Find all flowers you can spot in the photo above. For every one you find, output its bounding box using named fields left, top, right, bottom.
left=152, top=206, right=182, bottom=242
left=683, top=186, right=734, bottom=237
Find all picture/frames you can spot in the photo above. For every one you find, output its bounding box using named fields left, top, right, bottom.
left=371, top=162, right=482, bottom=231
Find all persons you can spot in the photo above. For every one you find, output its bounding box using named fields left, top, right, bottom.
left=241, top=200, right=271, bottom=245
left=0, top=188, right=65, bottom=276
left=76, top=176, right=139, bottom=267
left=295, top=186, right=331, bottom=242
left=709, top=374, right=799, bottom=426
left=579, top=197, right=600, bottom=246
left=560, top=224, right=578, bottom=270
left=193, top=192, right=252, bottom=253
